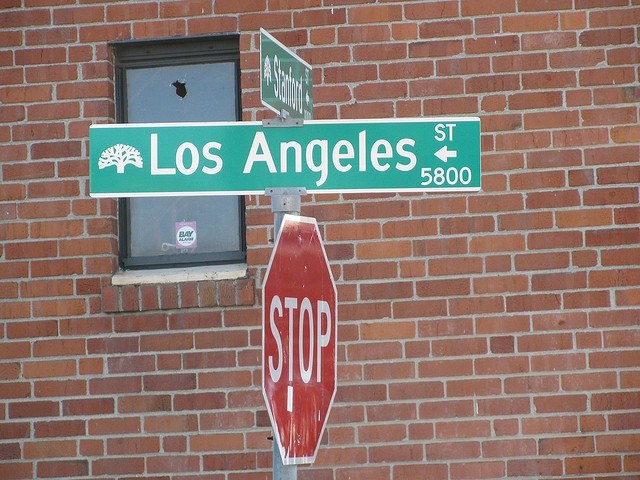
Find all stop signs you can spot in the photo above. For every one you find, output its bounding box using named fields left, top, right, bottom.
left=261, top=214, right=338, bottom=466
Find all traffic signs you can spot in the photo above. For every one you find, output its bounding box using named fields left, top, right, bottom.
left=260, top=26, right=313, bottom=118
left=89, top=117, right=481, bottom=198
